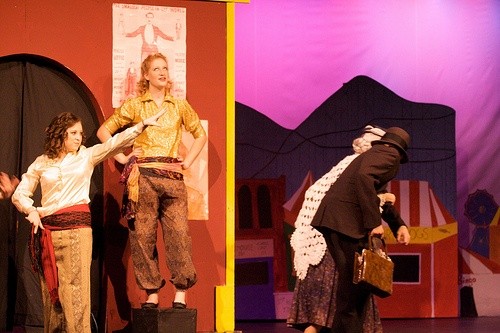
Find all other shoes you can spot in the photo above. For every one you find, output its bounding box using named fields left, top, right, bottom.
left=142, top=302, right=159, bottom=308
left=172, top=300, right=186, bottom=309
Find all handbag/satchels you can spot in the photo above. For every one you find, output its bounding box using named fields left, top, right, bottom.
left=352, top=233, right=394, bottom=298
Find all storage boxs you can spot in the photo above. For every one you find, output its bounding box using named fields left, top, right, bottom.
left=131, top=308, right=197, bottom=333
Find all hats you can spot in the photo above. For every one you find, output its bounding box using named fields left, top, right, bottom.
left=371, top=127, right=410, bottom=159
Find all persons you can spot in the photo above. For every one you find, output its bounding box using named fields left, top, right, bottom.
left=12, top=107, right=168, bottom=333
left=96, top=53, right=208, bottom=309
left=0, top=171, right=20, bottom=201
left=122, top=11, right=179, bottom=95
left=286, top=125, right=411, bottom=333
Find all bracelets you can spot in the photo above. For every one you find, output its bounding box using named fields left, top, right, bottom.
left=142, top=120, right=145, bottom=126
